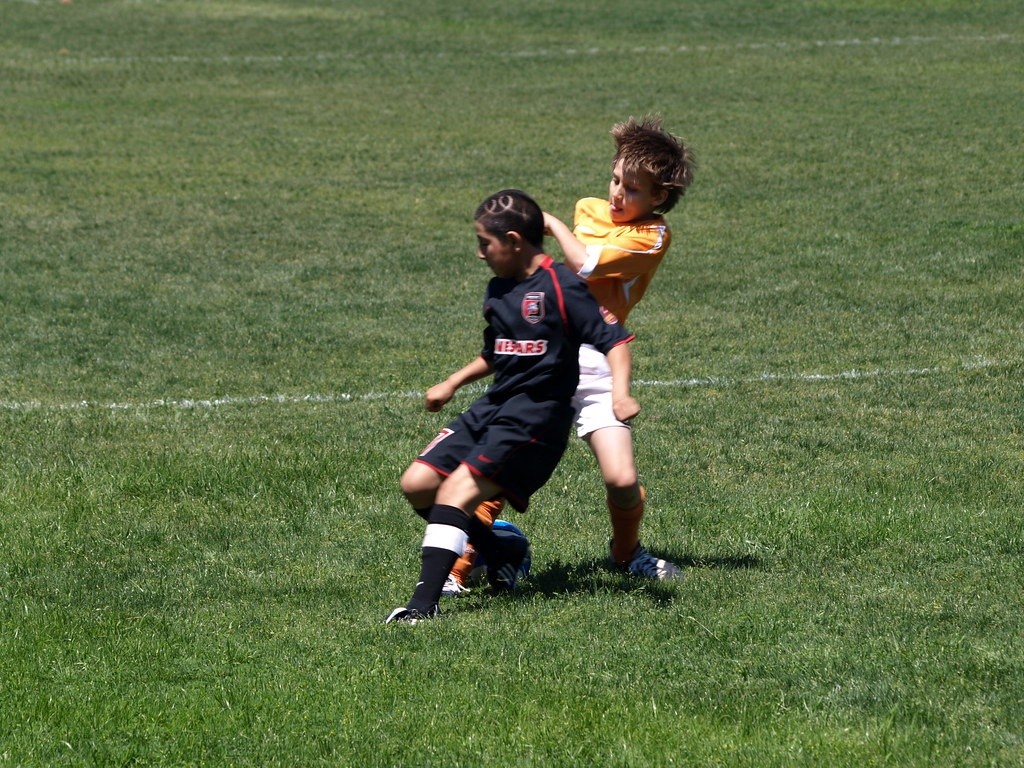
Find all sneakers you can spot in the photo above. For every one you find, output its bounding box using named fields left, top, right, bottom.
left=609, top=538, right=680, bottom=586
left=483, top=535, right=528, bottom=597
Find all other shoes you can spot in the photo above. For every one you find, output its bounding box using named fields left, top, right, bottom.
left=441, top=574, right=473, bottom=598
left=386, top=605, right=442, bottom=627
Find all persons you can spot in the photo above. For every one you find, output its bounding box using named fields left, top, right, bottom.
left=386, top=190, right=642, bottom=625
left=439, top=115, right=697, bottom=597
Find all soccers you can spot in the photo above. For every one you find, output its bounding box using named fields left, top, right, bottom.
left=469, top=519, right=532, bottom=585
